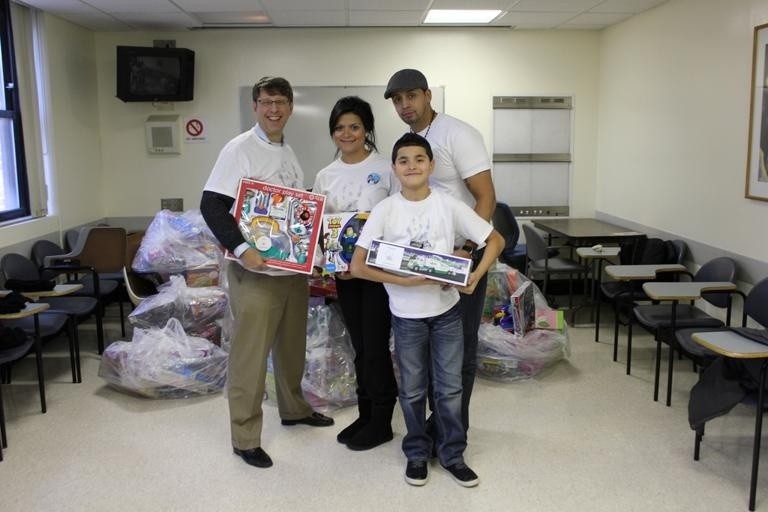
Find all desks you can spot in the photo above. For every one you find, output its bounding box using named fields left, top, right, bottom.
left=691, top=331, right=767, bottom=510
left=530, top=217, right=644, bottom=320
left=604, top=264, right=684, bottom=375
left=577, top=246, right=621, bottom=343
left=640, top=282, right=735, bottom=404
left=0, top=303, right=50, bottom=413
left=2, top=284, right=85, bottom=299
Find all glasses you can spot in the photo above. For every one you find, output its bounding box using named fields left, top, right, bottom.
left=257, top=99, right=289, bottom=107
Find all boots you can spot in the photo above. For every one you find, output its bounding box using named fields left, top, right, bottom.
left=337, top=389, right=397, bottom=451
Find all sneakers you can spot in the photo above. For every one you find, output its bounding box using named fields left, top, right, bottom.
left=405, top=459, right=431, bottom=486
left=438, top=458, right=479, bottom=487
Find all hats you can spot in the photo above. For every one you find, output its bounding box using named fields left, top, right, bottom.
left=384, top=69, right=428, bottom=99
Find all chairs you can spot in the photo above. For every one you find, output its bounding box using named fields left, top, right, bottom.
left=0, top=253, right=97, bottom=384
left=664, top=239, right=688, bottom=263
left=522, top=224, right=583, bottom=315
left=676, top=279, right=767, bottom=461
left=627, top=257, right=737, bottom=376
left=33, top=241, right=116, bottom=355
left=1, top=337, right=33, bottom=447
left=66, top=229, right=78, bottom=250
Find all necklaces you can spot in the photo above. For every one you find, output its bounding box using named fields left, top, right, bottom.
left=410, top=111, right=437, bottom=138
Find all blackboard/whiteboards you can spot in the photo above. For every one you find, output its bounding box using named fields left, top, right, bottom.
left=240, top=85, right=445, bottom=190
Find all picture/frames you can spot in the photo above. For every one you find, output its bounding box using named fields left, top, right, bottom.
left=745, top=23, right=768, bottom=201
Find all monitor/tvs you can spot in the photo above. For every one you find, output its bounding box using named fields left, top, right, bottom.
left=116, top=45, right=195, bottom=103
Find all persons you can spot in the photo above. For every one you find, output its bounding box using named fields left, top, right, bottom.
left=353, top=133, right=507, bottom=488
left=314, top=95, right=398, bottom=452
left=339, top=226, right=359, bottom=262
left=384, top=70, right=497, bottom=457
left=199, top=77, right=334, bottom=467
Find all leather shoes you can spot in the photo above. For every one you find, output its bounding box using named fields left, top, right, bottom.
left=282, top=412, right=334, bottom=426
left=233, top=446, right=273, bottom=468
